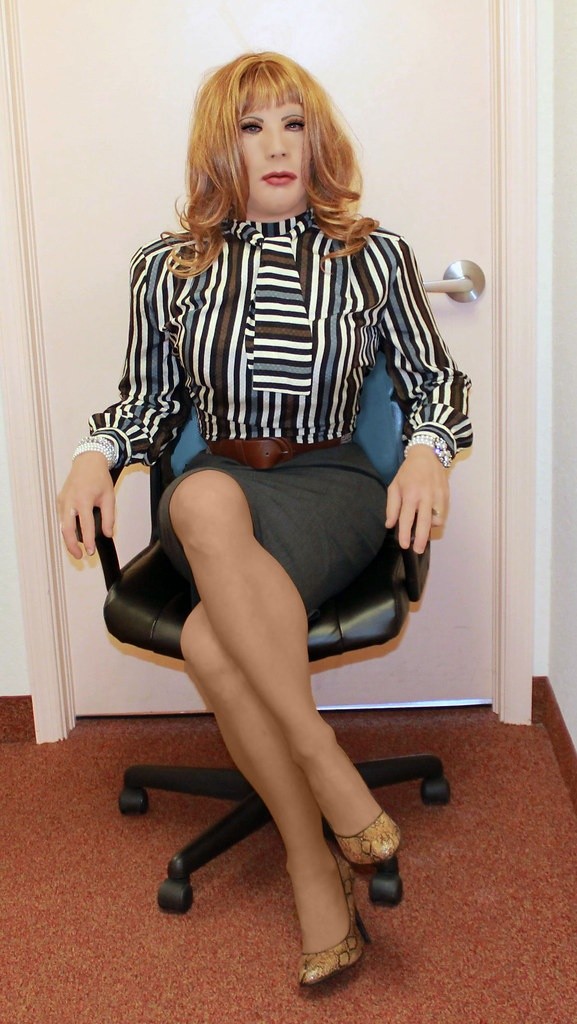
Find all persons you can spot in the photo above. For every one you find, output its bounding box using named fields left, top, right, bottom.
left=56, top=50, right=472, bottom=987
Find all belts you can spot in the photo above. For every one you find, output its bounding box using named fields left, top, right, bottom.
left=206, top=437, right=341, bottom=468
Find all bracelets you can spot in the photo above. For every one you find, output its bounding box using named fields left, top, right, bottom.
left=401, top=429, right=454, bottom=470
left=70, top=435, right=118, bottom=469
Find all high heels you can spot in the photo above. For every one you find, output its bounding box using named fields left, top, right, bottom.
left=326, top=744, right=401, bottom=865
left=295, top=851, right=370, bottom=985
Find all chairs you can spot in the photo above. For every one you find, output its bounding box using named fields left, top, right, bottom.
left=69, top=337, right=451, bottom=916
